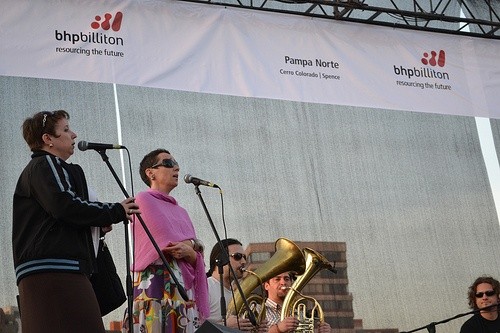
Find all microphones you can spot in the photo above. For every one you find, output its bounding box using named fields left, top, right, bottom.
left=77, top=140, right=127, bottom=152
left=184, top=174, right=219, bottom=188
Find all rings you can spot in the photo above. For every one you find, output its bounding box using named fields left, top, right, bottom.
left=129, top=209, right=133, bottom=214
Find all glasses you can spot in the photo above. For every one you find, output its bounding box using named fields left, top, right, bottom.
left=229, top=252, right=246, bottom=261
left=151, top=158, right=179, bottom=168
left=475, top=289, right=496, bottom=298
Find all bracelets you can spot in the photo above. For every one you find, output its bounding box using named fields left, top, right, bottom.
left=190, top=238, right=195, bottom=250
left=276, top=324, right=282, bottom=333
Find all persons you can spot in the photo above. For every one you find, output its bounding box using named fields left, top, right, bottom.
left=120, top=148, right=211, bottom=333
left=12, top=108, right=142, bottom=333
left=204, top=238, right=270, bottom=333
left=262, top=269, right=332, bottom=333
left=460, top=276, right=500, bottom=333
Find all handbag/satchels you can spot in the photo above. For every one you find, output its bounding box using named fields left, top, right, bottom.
left=89, top=235, right=126, bottom=318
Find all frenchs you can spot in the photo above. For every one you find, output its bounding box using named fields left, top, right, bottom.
left=226, top=237, right=334, bottom=333
